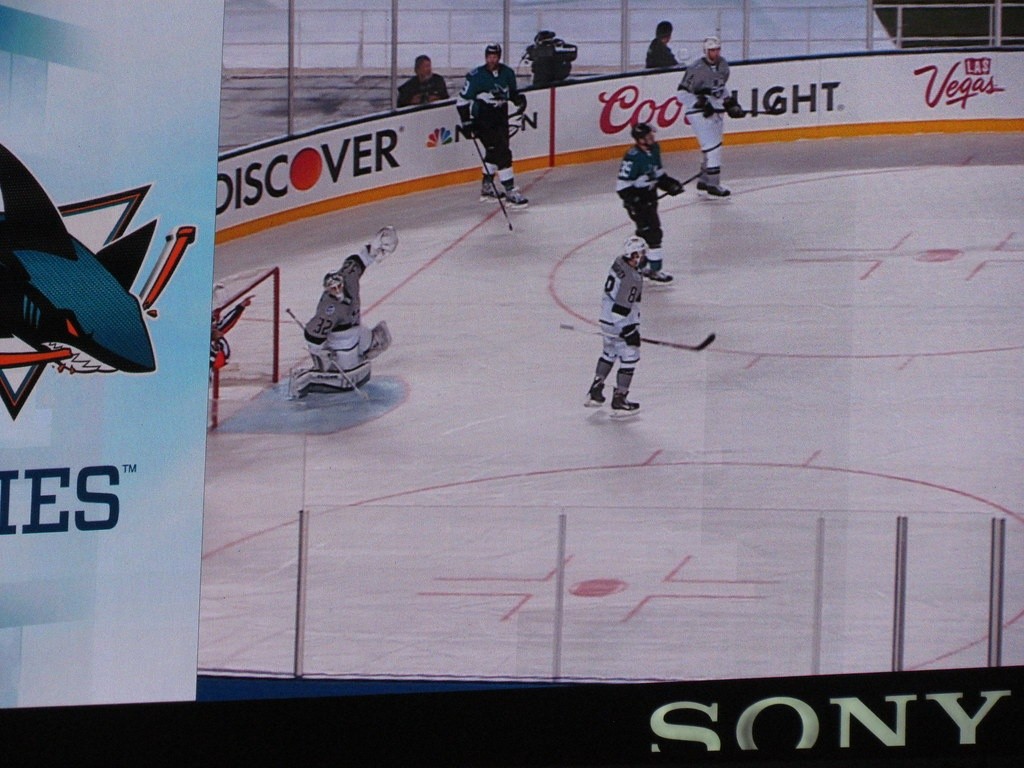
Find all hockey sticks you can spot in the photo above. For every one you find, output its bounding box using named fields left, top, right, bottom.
left=286, top=308, right=368, bottom=402
left=470, top=131, right=514, bottom=232
left=712, top=107, right=788, bottom=116
left=559, top=321, right=716, bottom=352
left=650, top=167, right=712, bottom=201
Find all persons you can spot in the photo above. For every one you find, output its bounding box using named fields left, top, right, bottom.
left=646, top=22, right=678, bottom=69
left=583, top=236, right=650, bottom=417
left=398, top=55, right=449, bottom=107
left=616, top=123, right=684, bottom=285
left=528, top=30, right=572, bottom=86
left=288, top=224, right=398, bottom=400
left=456, top=42, right=529, bottom=209
left=676, top=36, right=747, bottom=199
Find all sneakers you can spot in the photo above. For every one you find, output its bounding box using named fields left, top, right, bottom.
left=480, top=181, right=507, bottom=202
left=504, top=187, right=529, bottom=208
left=584, top=377, right=606, bottom=406
left=611, top=388, right=640, bottom=417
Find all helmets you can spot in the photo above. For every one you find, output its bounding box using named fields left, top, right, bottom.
left=702, top=36, right=721, bottom=54
left=485, top=43, right=502, bottom=59
left=631, top=123, right=652, bottom=146
left=323, top=272, right=345, bottom=299
left=624, top=235, right=649, bottom=258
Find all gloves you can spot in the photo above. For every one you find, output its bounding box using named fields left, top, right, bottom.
left=634, top=193, right=657, bottom=214
left=694, top=97, right=714, bottom=118
left=622, top=324, right=640, bottom=347
left=461, top=119, right=478, bottom=139
left=512, top=94, right=526, bottom=106
left=723, top=96, right=745, bottom=118
left=657, top=176, right=685, bottom=196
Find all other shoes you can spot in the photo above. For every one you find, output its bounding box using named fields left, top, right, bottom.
left=638, top=268, right=651, bottom=281
left=289, top=368, right=299, bottom=398
left=696, top=181, right=708, bottom=194
left=707, top=184, right=730, bottom=200
left=648, top=269, right=673, bottom=285
